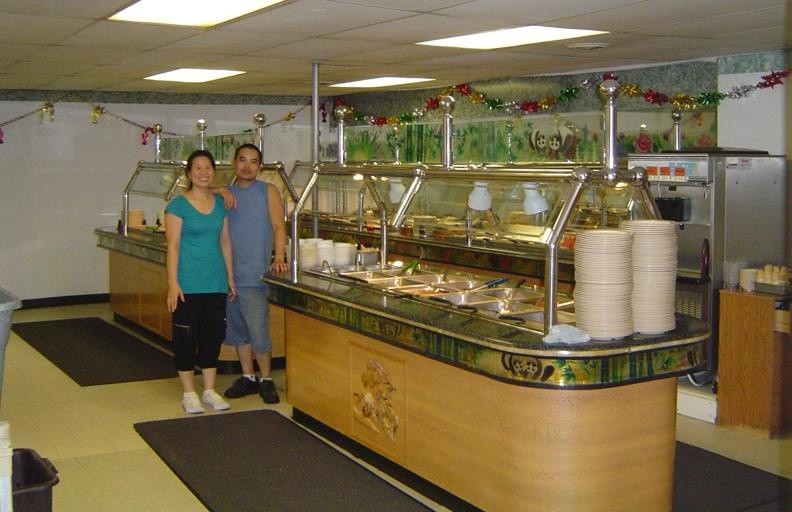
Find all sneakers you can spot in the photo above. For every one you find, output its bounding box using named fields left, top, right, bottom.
left=202, top=391, right=231, bottom=410
left=224, top=376, right=260, bottom=398
left=181, top=395, right=205, bottom=413
left=260, top=380, right=280, bottom=403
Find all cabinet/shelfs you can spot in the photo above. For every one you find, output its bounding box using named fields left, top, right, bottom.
left=262, top=162, right=664, bottom=339
left=122, top=161, right=302, bottom=338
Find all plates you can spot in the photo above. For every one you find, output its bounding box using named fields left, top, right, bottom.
left=737, top=267, right=756, bottom=292
left=572, top=219, right=678, bottom=344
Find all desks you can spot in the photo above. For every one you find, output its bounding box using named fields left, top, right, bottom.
left=713, top=288, right=792, bottom=440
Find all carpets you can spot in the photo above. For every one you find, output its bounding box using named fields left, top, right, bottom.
left=132, top=405, right=438, bottom=512
left=8, top=315, right=203, bottom=388
left=673, top=439, right=791, bottom=512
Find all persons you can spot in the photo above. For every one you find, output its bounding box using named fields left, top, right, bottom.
left=212, top=141, right=291, bottom=405
left=164, top=147, right=237, bottom=415
